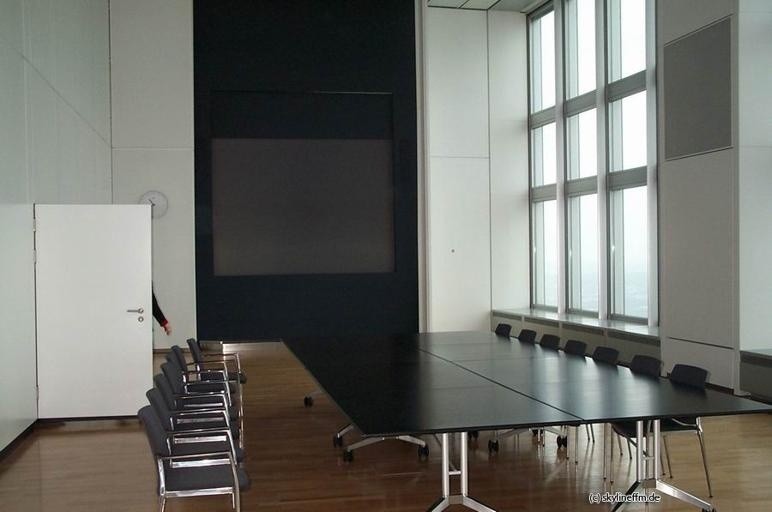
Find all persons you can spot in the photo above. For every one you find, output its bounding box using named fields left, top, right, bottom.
left=152, top=284, right=172, bottom=335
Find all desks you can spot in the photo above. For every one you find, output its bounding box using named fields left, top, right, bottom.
left=281, top=331, right=771, bottom=512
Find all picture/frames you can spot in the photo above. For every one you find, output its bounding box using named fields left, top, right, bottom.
left=665, top=13, right=735, bottom=161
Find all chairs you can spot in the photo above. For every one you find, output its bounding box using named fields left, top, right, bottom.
left=541, top=334, right=559, bottom=349
left=586, top=346, right=714, bottom=499
left=564, top=340, right=586, bottom=356
left=495, top=323, right=512, bottom=336
left=519, top=330, right=536, bottom=343
left=136, top=338, right=248, bottom=512
